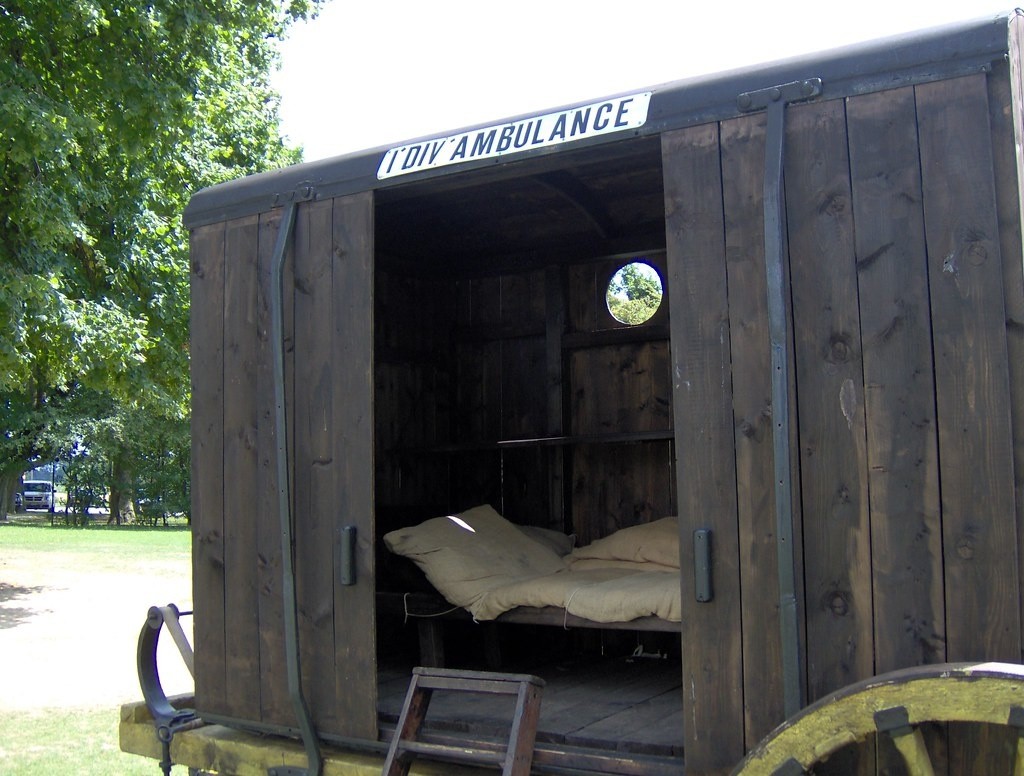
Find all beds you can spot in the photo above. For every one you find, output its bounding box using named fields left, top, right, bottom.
left=375, top=515, right=681, bottom=668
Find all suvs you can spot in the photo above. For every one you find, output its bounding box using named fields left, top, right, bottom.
left=14, top=479, right=57, bottom=513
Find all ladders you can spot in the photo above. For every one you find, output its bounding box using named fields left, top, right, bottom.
left=379, top=664, right=547, bottom=776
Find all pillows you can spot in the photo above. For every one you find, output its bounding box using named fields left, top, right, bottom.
left=383, top=504, right=566, bottom=607
